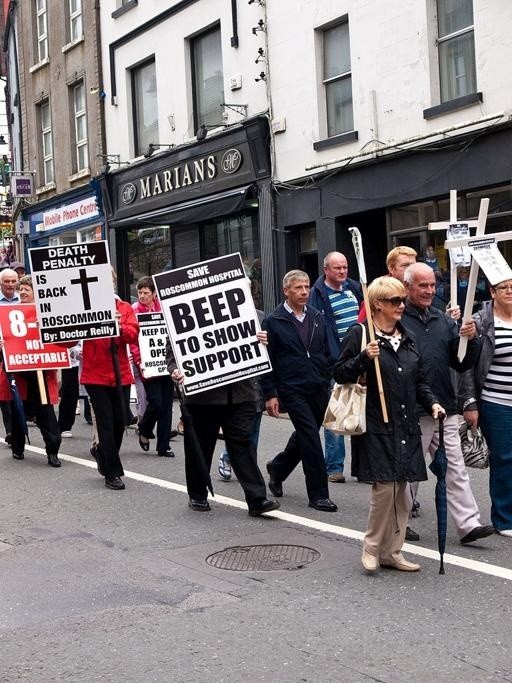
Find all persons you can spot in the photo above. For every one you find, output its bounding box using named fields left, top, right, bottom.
left=307, top=251, right=365, bottom=484
left=130, top=276, right=174, bottom=458
left=177, top=415, right=185, bottom=436
left=9, top=261, right=26, bottom=280
left=58, top=342, right=93, bottom=437
left=357, top=246, right=416, bottom=325
left=0, top=274, right=62, bottom=467
left=55, top=265, right=140, bottom=491
left=0, top=268, right=21, bottom=446
left=134, top=362, right=177, bottom=438
left=261, top=267, right=338, bottom=513
left=402, top=263, right=494, bottom=547
left=218, top=308, right=264, bottom=480
left=165, top=330, right=279, bottom=518
left=460, top=277, right=512, bottom=535
left=73, top=341, right=100, bottom=457
left=332, top=275, right=448, bottom=573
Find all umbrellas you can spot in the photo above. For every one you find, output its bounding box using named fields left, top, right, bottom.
left=173, top=377, right=214, bottom=497
left=428, top=411, right=449, bottom=575
left=2, top=363, right=32, bottom=445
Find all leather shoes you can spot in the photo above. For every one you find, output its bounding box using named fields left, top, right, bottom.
left=138, top=431, right=150, bottom=451
left=266, top=460, right=283, bottom=497
left=460, top=526, right=496, bottom=544
left=361, top=541, right=378, bottom=570
left=328, top=473, right=345, bottom=483
left=248, top=499, right=281, bottom=516
left=308, top=496, right=337, bottom=511
left=187, top=495, right=210, bottom=511
left=46, top=452, right=62, bottom=467
left=405, top=526, right=420, bottom=541
left=12, top=452, right=25, bottom=460
left=379, top=557, right=421, bottom=572
left=176, top=424, right=184, bottom=436
left=157, top=451, right=174, bottom=457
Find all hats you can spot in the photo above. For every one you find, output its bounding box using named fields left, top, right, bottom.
left=9, top=262, right=26, bottom=271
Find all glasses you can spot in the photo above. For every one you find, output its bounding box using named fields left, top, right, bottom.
left=377, top=295, right=408, bottom=306
left=494, top=286, right=512, bottom=292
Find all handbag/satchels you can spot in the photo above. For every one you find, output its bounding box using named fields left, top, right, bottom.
left=321, top=322, right=368, bottom=435
left=458, top=397, right=491, bottom=470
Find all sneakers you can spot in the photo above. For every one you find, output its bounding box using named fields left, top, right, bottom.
left=60, top=430, right=73, bottom=438
left=89, top=444, right=105, bottom=476
left=499, top=529, right=512, bottom=537
left=103, top=475, right=125, bottom=490
left=218, top=452, right=232, bottom=481
